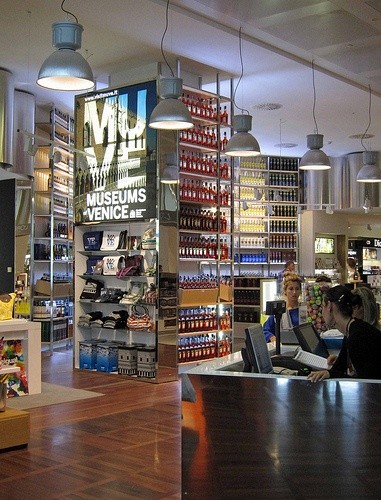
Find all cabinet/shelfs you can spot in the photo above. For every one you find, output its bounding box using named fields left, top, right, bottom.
left=225, top=155, right=302, bottom=265
left=234, top=277, right=273, bottom=339
left=32, top=105, right=76, bottom=356
left=73, top=217, right=159, bottom=383
left=177, top=83, right=234, bottom=368
left=0, top=365, right=30, bottom=450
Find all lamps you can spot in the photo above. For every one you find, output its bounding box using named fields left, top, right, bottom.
left=224, top=26, right=261, bottom=157
left=160, top=150, right=179, bottom=185
left=299, top=59, right=332, bottom=170
left=326, top=205, right=333, bottom=216
left=356, top=84, right=381, bottom=183
left=362, top=196, right=373, bottom=211
left=241, top=189, right=282, bottom=218
left=21, top=134, right=37, bottom=156
left=148, top=0, right=194, bottom=131
left=36, top=0, right=94, bottom=91
left=47, top=144, right=62, bottom=164
left=296, top=206, right=302, bottom=214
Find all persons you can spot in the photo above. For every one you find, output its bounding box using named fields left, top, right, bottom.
left=346, top=258, right=360, bottom=282
left=284, top=261, right=294, bottom=272
left=327, top=287, right=381, bottom=377
left=307, top=285, right=381, bottom=383
left=262, top=273, right=303, bottom=342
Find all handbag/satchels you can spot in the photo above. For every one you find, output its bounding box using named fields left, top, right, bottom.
left=127, top=305, right=154, bottom=333
left=0, top=289, right=16, bottom=321
left=80, top=230, right=157, bottom=306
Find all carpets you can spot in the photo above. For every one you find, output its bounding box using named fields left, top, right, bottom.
left=7, top=382, right=107, bottom=410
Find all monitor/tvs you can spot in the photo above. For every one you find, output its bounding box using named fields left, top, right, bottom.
left=240, top=324, right=274, bottom=374
left=292, top=321, right=329, bottom=360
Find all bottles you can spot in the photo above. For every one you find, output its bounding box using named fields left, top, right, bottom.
left=178, top=306, right=231, bottom=333
left=180, top=274, right=232, bottom=289
left=180, top=207, right=228, bottom=234
left=33, top=109, right=74, bottom=340
left=180, top=150, right=229, bottom=178
left=177, top=333, right=231, bottom=362
left=179, top=125, right=230, bottom=150
left=234, top=156, right=298, bottom=322
left=179, top=178, right=228, bottom=207
left=181, top=94, right=228, bottom=124
left=180, top=236, right=229, bottom=260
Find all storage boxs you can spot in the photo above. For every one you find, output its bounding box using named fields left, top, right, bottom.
left=34, top=280, right=73, bottom=297
left=177, top=285, right=232, bottom=304
left=78, top=339, right=156, bottom=379
